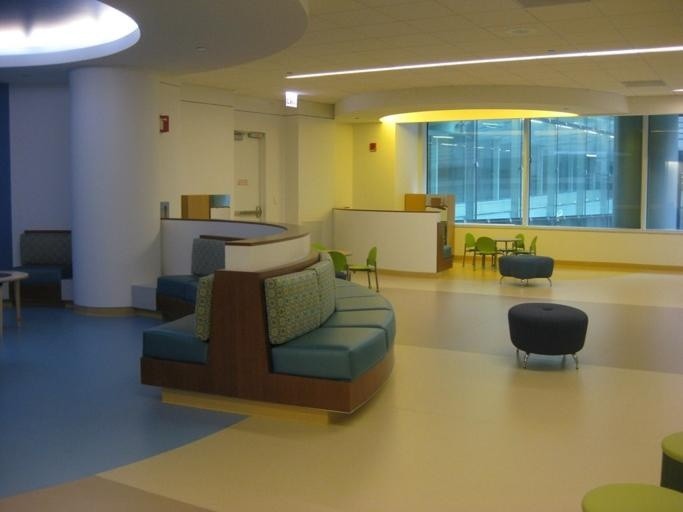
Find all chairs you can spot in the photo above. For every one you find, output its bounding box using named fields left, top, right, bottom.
left=463, top=232, right=538, bottom=273
left=310, top=243, right=379, bottom=293
left=581, top=481, right=681, bottom=512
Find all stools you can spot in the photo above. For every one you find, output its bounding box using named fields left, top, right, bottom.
left=508, top=303, right=588, bottom=370
left=661, top=430, right=682, bottom=493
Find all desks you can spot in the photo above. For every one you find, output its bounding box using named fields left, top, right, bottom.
left=0, top=271, right=29, bottom=334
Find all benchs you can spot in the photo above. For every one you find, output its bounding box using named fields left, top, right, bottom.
left=444, top=245, right=451, bottom=270
left=499, top=256, right=554, bottom=288
left=9, top=229, right=72, bottom=308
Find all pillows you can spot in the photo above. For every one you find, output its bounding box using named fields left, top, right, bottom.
left=319, top=251, right=332, bottom=262
left=193, top=273, right=213, bottom=341
left=306, top=260, right=336, bottom=324
left=264, top=270, right=320, bottom=344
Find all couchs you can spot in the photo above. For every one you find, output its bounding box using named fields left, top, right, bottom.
left=141, top=251, right=396, bottom=423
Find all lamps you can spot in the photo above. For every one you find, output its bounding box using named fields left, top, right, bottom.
left=285, top=91, right=298, bottom=108
